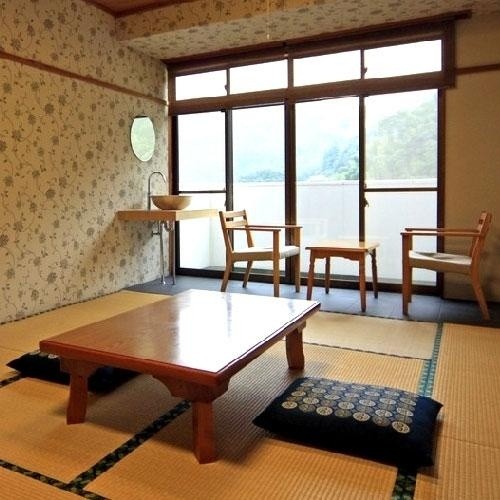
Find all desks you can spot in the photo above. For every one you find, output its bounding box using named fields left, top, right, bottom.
left=305, top=239, right=380, bottom=313
left=40, top=288, right=321, bottom=463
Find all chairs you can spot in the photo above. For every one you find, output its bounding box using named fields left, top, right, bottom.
left=401, top=211, right=492, bottom=321
left=220, top=210, right=303, bottom=297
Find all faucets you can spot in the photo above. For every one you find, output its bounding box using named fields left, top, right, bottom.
left=147, top=171, right=167, bottom=211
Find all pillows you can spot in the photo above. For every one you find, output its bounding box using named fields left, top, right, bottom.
left=131, top=115, right=156, bottom=162
left=253, top=378, right=444, bottom=473
left=7, top=350, right=145, bottom=397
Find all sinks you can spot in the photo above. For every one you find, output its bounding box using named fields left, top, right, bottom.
left=150, top=194, right=193, bottom=210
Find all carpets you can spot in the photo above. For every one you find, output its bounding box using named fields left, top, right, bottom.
left=282, top=309, right=439, bottom=361
left=126, top=275, right=500, bottom=328
